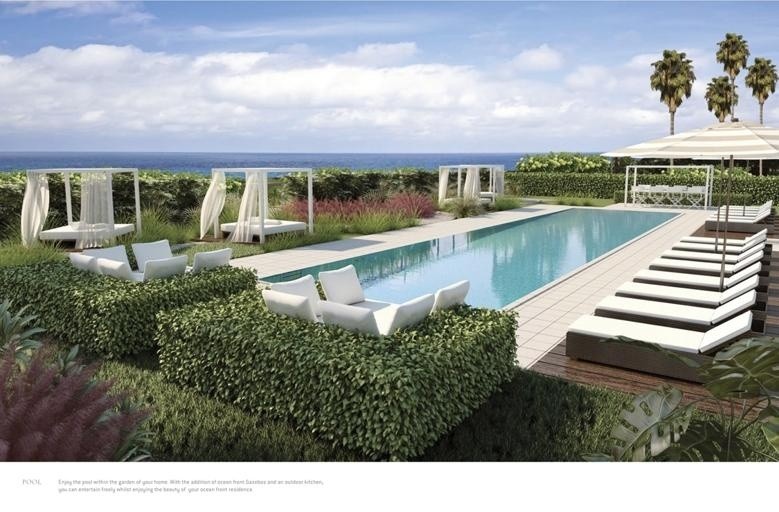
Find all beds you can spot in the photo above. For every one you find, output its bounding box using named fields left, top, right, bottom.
left=220, top=215, right=307, bottom=244
left=41, top=220, right=135, bottom=249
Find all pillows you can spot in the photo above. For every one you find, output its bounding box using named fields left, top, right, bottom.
left=263, top=266, right=471, bottom=333
left=68, top=240, right=232, bottom=281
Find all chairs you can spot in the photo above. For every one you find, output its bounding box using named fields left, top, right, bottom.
left=630, top=182, right=706, bottom=210
left=563, top=228, right=770, bottom=384
left=702, top=200, right=778, bottom=234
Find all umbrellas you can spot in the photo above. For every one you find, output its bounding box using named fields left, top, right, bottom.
left=599, top=117, right=779, bottom=293
